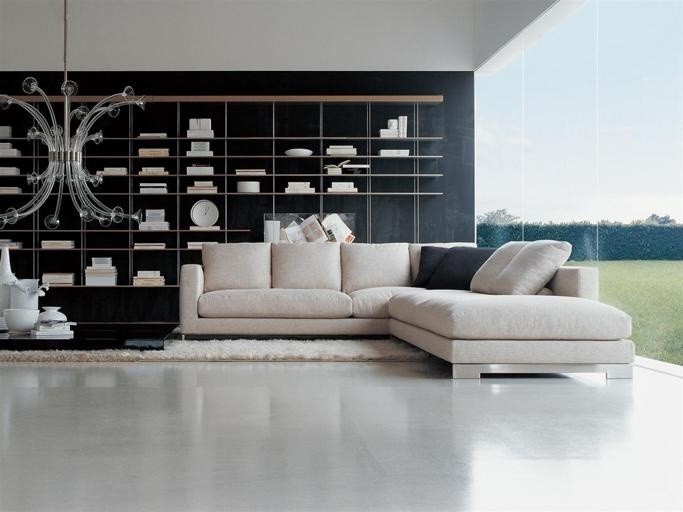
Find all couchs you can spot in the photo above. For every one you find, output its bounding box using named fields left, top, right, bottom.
left=176, top=239, right=641, bottom=383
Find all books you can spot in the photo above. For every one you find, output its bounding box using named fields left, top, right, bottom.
left=29, top=321, right=77, bottom=339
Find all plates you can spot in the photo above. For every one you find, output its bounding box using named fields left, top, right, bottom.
left=284, top=148, right=314, bottom=157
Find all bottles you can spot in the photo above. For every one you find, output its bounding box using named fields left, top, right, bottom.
left=0, top=244, right=18, bottom=330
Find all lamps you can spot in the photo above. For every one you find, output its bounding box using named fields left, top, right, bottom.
left=1, top=1, right=147, bottom=231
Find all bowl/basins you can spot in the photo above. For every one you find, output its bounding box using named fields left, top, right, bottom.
left=2, top=309, right=41, bottom=335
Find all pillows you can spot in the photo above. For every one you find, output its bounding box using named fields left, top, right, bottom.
left=409, top=240, right=573, bottom=296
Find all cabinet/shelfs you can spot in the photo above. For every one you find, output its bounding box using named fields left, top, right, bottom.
left=0, top=94, right=445, bottom=336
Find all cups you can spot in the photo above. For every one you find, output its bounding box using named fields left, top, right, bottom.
left=10, top=278, right=39, bottom=309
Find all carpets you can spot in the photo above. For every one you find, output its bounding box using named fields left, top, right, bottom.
left=0, top=335, right=429, bottom=364
